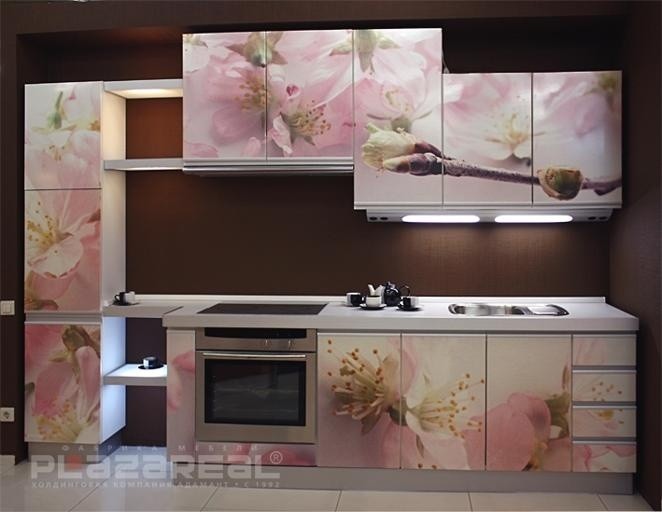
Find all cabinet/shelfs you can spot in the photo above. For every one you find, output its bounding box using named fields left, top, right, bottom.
left=24, top=28, right=638, bottom=494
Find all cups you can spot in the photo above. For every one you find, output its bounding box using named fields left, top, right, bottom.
left=365, top=295, right=382, bottom=306
left=115, top=290, right=137, bottom=304
left=399, top=296, right=419, bottom=307
left=143, top=356, right=158, bottom=366
left=346, top=291, right=365, bottom=306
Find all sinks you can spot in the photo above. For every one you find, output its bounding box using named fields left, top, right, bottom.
left=448, top=301, right=523, bottom=317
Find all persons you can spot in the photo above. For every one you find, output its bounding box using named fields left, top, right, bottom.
left=57, top=324, right=101, bottom=410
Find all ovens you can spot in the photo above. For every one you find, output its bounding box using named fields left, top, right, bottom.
left=190, top=349, right=316, bottom=446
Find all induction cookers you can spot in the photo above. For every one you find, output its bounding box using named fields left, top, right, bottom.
left=195, top=303, right=326, bottom=351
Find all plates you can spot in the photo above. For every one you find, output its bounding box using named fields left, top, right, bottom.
left=399, top=304, right=423, bottom=310
left=138, top=362, right=166, bottom=370
left=360, top=304, right=386, bottom=309
left=113, top=300, right=139, bottom=306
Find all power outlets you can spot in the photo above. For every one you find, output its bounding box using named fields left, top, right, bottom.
left=0, top=407, right=15, bottom=422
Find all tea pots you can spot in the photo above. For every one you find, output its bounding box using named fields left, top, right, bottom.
left=367, top=281, right=411, bottom=305
